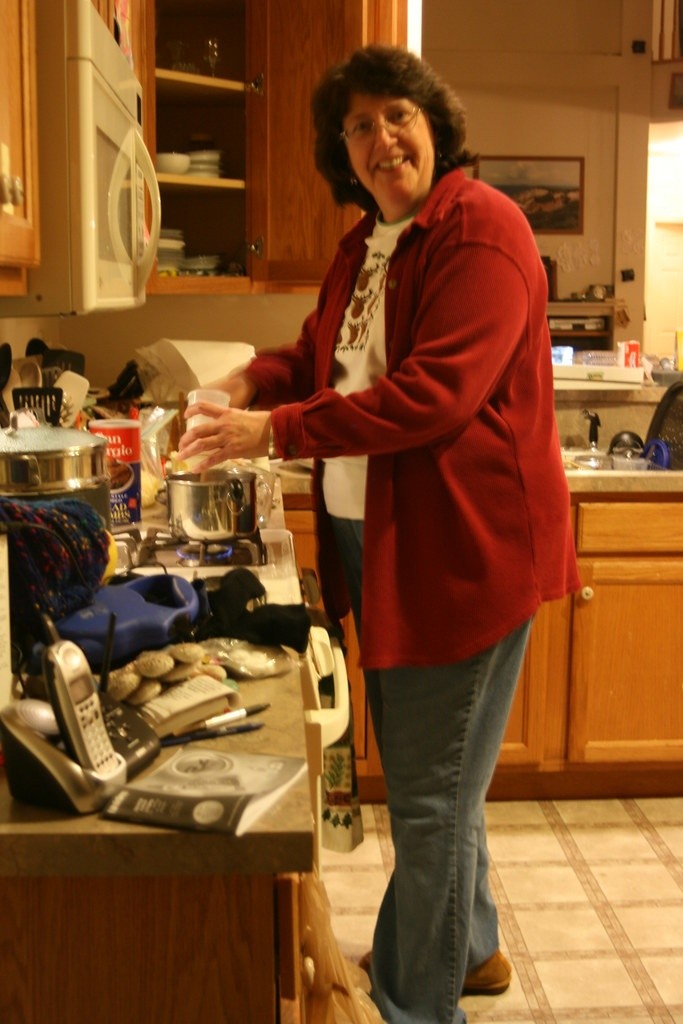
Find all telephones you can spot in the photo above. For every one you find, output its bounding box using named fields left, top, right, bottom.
left=0, top=613, right=127, bottom=813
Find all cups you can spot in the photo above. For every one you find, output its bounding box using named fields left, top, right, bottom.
left=571, top=284, right=614, bottom=301
left=184, top=390, right=230, bottom=470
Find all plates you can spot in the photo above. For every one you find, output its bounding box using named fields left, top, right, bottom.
left=156, top=229, right=221, bottom=277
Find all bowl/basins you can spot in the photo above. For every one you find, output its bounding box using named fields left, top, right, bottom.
left=558, top=448, right=650, bottom=472
left=158, top=149, right=222, bottom=177
left=651, top=369, right=683, bottom=386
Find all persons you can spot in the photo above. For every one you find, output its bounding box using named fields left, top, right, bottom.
left=175, top=44, right=582, bottom=1024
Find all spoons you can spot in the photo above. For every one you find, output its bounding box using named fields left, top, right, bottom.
left=0, top=337, right=50, bottom=427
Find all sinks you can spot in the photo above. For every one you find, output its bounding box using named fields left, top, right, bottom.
left=560, top=453, right=667, bottom=473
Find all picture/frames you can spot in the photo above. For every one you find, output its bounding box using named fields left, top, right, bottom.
left=475, top=155, right=586, bottom=237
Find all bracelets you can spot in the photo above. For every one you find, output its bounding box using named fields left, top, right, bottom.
left=268, top=426, right=275, bottom=460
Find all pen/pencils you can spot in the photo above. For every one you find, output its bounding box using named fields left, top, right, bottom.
left=166, top=702, right=272, bottom=745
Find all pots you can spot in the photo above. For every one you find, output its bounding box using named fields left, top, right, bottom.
left=0, top=428, right=113, bottom=540
left=166, top=468, right=257, bottom=542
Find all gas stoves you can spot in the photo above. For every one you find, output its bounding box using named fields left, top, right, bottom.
left=95, top=522, right=304, bottom=606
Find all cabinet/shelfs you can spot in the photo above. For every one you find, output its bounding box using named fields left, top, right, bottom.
left=95, top=0, right=408, bottom=295
left=0, top=0, right=42, bottom=297
left=282, top=492, right=683, bottom=803
left=0, top=870, right=310, bottom=1023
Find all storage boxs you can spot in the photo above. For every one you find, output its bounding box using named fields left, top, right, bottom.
left=553, top=365, right=644, bottom=390
left=617, top=341, right=640, bottom=366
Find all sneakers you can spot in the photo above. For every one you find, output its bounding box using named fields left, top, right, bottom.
left=361, top=945, right=514, bottom=997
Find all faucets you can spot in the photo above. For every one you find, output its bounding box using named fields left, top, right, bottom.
left=583, top=408, right=602, bottom=450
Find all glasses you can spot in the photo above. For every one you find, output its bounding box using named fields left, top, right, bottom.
left=341, top=106, right=424, bottom=142
